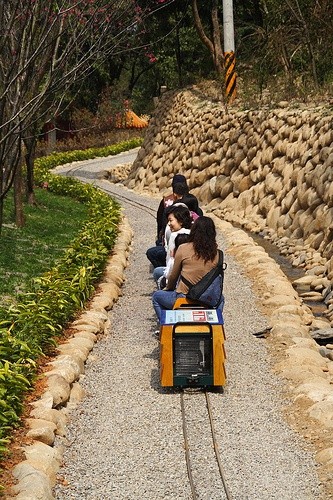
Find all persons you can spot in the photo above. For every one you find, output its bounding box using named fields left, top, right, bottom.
left=146, top=174, right=225, bottom=336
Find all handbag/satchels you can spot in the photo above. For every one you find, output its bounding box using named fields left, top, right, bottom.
left=180, top=249, right=227, bottom=309
